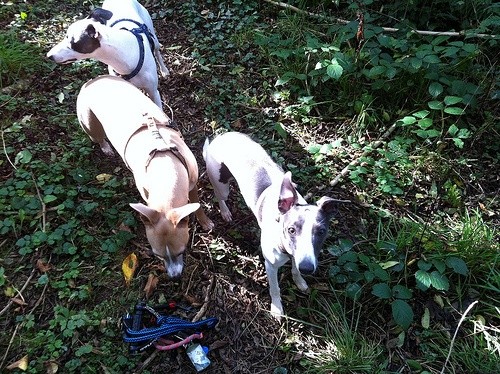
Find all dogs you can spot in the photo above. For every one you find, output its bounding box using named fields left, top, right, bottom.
left=45, top=0, right=169, bottom=111
left=75, top=74, right=215, bottom=278
left=201, top=131, right=352, bottom=325
